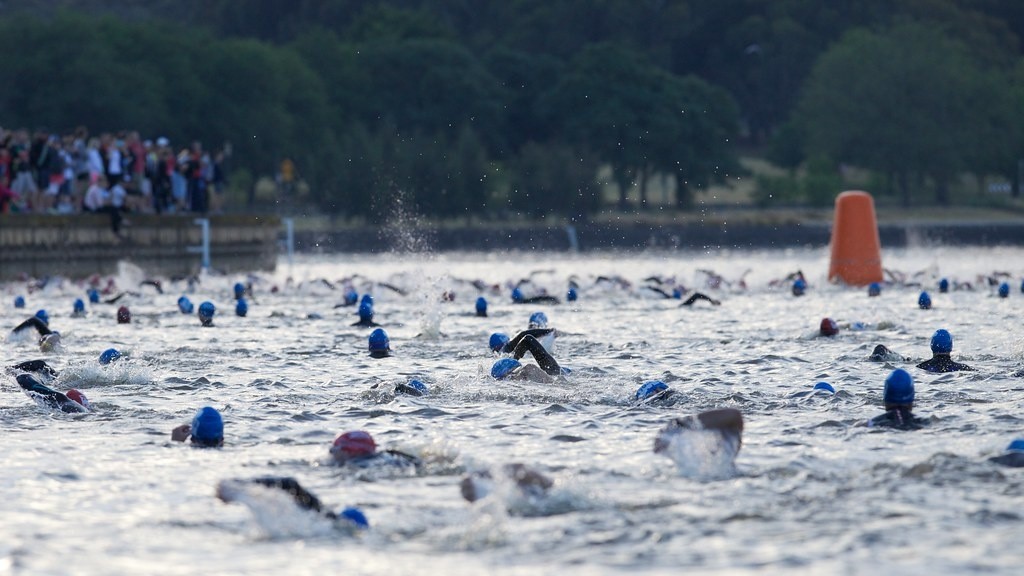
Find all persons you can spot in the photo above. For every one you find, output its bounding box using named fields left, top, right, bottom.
left=215, top=477, right=367, bottom=526
left=1, top=130, right=224, bottom=239
left=989, top=438, right=1024, bottom=467
left=870, top=369, right=930, bottom=432
left=368, top=328, right=392, bottom=359
left=39, top=333, right=59, bottom=352
left=916, top=328, right=977, bottom=373
left=490, top=328, right=557, bottom=353
left=6, top=348, right=122, bottom=379
left=657, top=409, right=743, bottom=460
left=460, top=463, right=553, bottom=501
left=330, top=432, right=418, bottom=467
left=514, top=334, right=571, bottom=376
left=490, top=359, right=555, bottom=384
left=395, top=379, right=426, bottom=396
left=172, top=406, right=225, bottom=447
left=529, top=312, right=582, bottom=336
left=16, top=373, right=91, bottom=414
left=0, top=270, right=1024, bottom=337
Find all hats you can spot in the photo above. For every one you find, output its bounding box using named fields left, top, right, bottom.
left=885, top=368, right=914, bottom=408
left=930, top=328, right=952, bottom=353
left=10, top=271, right=581, bottom=329
left=815, top=382, right=834, bottom=391
left=790, top=276, right=1024, bottom=307
left=636, top=380, right=667, bottom=398
left=369, top=329, right=389, bottom=350
left=1007, top=439, right=1024, bottom=450
left=333, top=431, right=375, bottom=455
left=343, top=507, right=371, bottom=528
left=409, top=379, right=428, bottom=394
left=820, top=317, right=839, bottom=337
left=190, top=408, right=223, bottom=440
left=490, top=358, right=520, bottom=380
left=99, top=348, right=121, bottom=365
left=67, top=390, right=88, bottom=408
